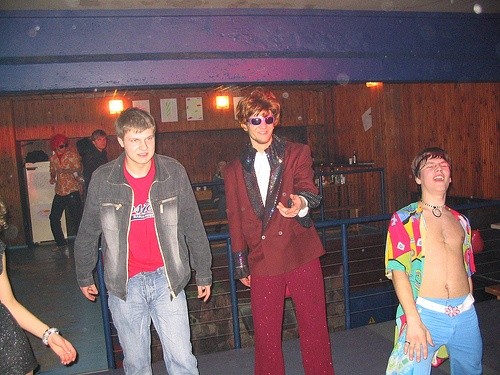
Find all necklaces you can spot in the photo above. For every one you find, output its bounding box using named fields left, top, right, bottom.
left=417, top=198, right=449, bottom=219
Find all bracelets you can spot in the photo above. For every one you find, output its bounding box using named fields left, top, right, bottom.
left=42, top=326, right=59, bottom=346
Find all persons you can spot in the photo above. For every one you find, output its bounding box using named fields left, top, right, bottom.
left=222, top=86, right=335, bottom=374
left=0, top=192, right=78, bottom=375
left=73, top=105, right=213, bottom=375
left=383, top=146, right=484, bottom=375
left=47, top=133, right=87, bottom=253
left=75, top=128, right=112, bottom=193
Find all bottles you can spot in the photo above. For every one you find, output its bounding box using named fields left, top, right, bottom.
left=319, top=151, right=358, bottom=184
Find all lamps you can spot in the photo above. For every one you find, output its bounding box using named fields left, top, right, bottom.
left=107, top=100, right=124, bottom=116
left=214, top=90, right=230, bottom=111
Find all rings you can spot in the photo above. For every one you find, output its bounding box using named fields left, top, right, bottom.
left=404, top=341, right=410, bottom=345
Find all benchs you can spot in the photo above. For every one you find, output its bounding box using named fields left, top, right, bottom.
left=194, top=186, right=365, bottom=248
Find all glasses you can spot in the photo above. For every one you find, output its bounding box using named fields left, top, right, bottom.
left=241, top=116, right=275, bottom=125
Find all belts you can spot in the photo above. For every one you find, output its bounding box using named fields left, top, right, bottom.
left=415, top=293, right=475, bottom=317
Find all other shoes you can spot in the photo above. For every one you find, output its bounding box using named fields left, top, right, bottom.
left=215, top=225, right=223, bottom=233
